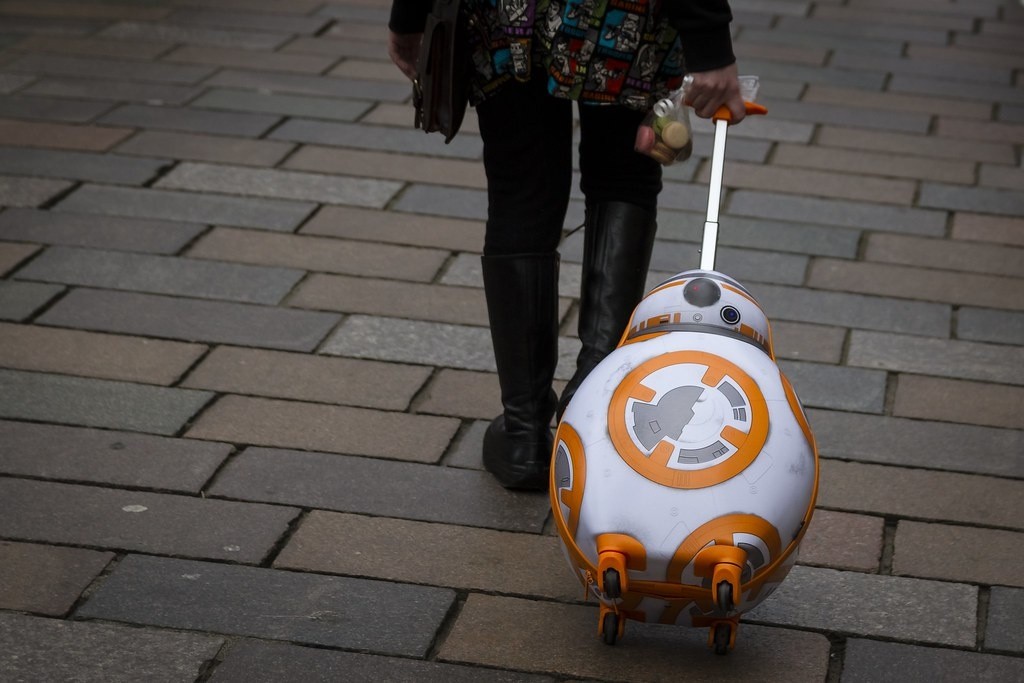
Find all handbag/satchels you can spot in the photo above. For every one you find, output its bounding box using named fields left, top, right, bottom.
left=412, top=0, right=471, bottom=145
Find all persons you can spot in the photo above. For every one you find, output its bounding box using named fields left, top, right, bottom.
left=389, top=0, right=746, bottom=490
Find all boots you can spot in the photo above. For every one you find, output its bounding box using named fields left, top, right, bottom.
left=558, top=198, right=658, bottom=424
left=483, top=250, right=561, bottom=495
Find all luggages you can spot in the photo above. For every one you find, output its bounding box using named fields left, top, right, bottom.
left=552, top=102, right=819, bottom=650
